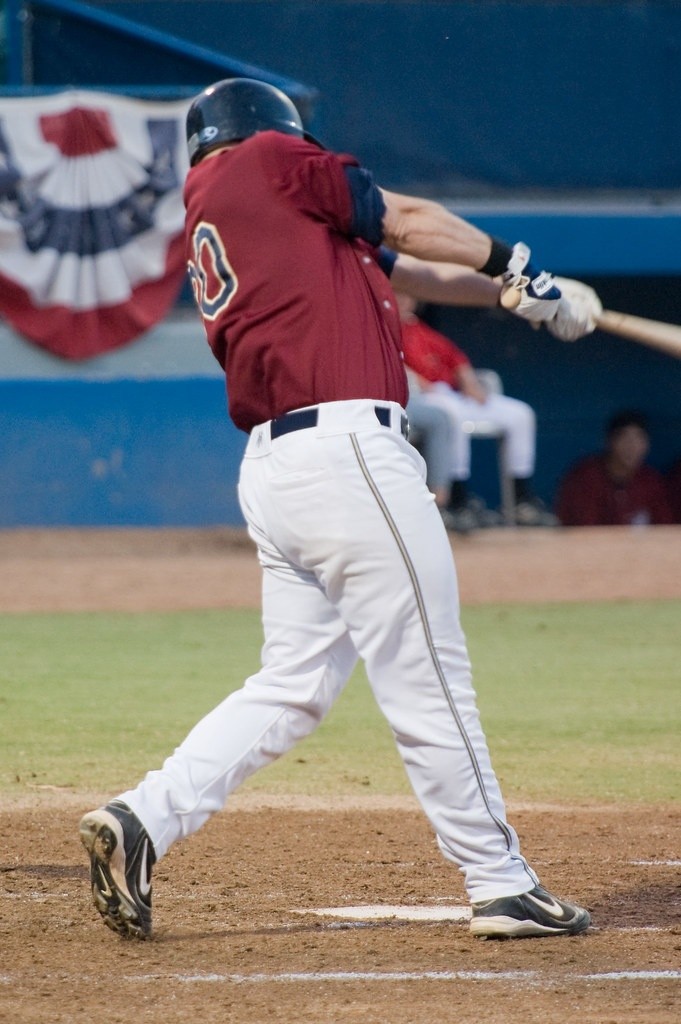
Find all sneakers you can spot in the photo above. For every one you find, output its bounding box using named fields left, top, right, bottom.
left=471, top=882, right=593, bottom=937
left=79, top=799, right=156, bottom=941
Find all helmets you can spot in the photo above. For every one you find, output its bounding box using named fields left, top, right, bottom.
left=183, top=77, right=327, bottom=167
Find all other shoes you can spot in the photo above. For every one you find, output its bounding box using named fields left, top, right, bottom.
left=439, top=502, right=560, bottom=534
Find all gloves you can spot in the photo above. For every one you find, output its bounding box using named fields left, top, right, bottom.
left=549, top=264, right=605, bottom=345
left=502, top=239, right=561, bottom=323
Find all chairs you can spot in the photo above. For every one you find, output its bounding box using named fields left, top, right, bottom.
left=406, top=421, right=519, bottom=531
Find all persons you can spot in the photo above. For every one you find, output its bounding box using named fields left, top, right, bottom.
left=555, top=413, right=675, bottom=525
left=77, top=78, right=602, bottom=938
left=398, top=292, right=558, bottom=531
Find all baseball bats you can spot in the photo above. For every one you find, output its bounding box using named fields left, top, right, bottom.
left=502, top=281, right=680, bottom=351
